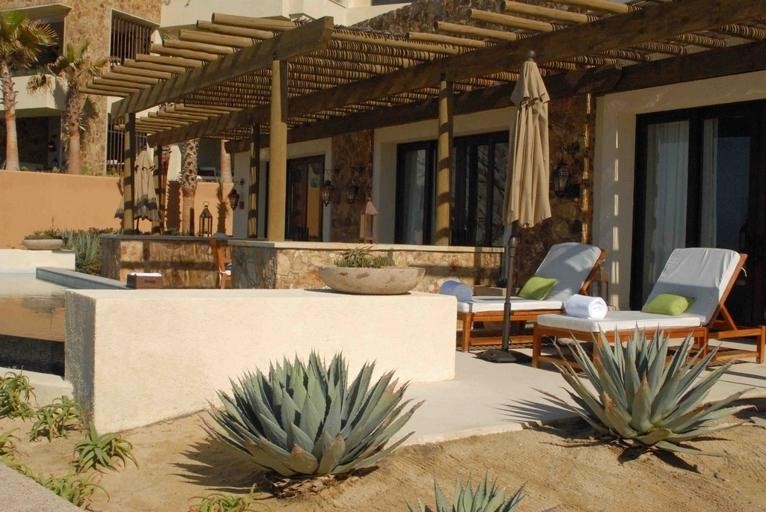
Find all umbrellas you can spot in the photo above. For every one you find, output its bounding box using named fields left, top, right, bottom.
left=114, top=146, right=160, bottom=232
left=497, top=49, right=553, bottom=349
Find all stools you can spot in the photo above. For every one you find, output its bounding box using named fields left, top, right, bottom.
left=127, top=271, right=163, bottom=290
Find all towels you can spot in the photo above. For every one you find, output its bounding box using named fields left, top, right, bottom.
left=440, top=279, right=470, bottom=302
left=563, top=291, right=607, bottom=322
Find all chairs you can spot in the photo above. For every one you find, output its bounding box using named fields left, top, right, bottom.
left=532, top=241, right=764, bottom=367
left=211, top=236, right=234, bottom=289
left=444, top=240, right=611, bottom=353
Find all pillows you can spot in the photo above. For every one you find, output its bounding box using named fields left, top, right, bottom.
left=520, top=277, right=559, bottom=303
left=641, top=291, right=695, bottom=315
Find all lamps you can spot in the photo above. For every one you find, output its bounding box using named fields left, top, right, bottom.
left=552, top=145, right=573, bottom=197
left=320, top=176, right=337, bottom=208
left=227, top=177, right=244, bottom=209
left=198, top=198, right=213, bottom=237
left=344, top=172, right=360, bottom=205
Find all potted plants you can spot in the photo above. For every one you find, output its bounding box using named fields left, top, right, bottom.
left=323, top=252, right=425, bottom=295
left=19, top=229, right=66, bottom=250
